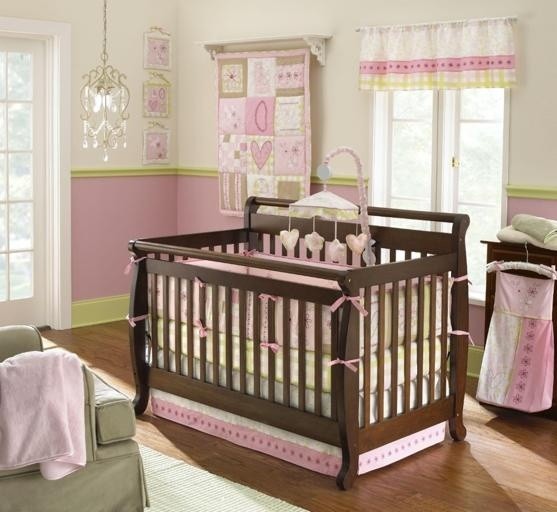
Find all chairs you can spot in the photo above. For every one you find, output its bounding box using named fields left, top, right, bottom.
left=0, top=324, right=150, bottom=512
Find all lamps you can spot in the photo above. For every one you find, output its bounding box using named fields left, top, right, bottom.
left=78, top=0, right=128, bottom=163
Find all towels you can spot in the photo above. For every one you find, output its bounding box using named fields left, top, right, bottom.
left=0, top=348, right=86, bottom=483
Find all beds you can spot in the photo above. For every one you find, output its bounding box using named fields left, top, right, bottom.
left=125, top=196, right=470, bottom=490
